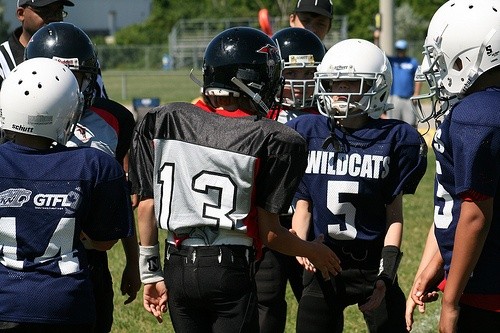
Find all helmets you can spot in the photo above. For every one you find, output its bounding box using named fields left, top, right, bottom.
left=204, top=27, right=283, bottom=116
left=317, top=38, right=394, bottom=119
left=421, top=0, right=500, bottom=110
left=271, top=27, right=326, bottom=68
left=394, top=40, right=409, bottom=50
left=0, top=57, right=84, bottom=146
left=25, top=22, right=101, bottom=74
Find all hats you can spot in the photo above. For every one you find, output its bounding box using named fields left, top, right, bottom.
left=16, top=0, right=74, bottom=8
left=295, top=0, right=333, bottom=19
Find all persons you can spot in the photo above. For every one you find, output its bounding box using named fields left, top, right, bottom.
left=412, top=0, right=500, bottom=333
left=0, top=0, right=439, bottom=333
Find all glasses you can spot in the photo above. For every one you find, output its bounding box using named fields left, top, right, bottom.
left=27, top=6, right=68, bottom=18
